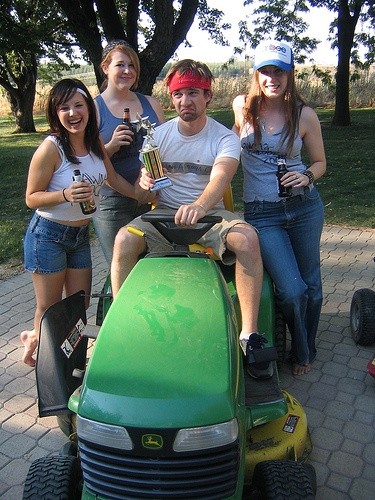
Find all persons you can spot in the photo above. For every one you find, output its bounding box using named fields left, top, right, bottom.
left=232, top=41, right=327, bottom=377
left=21, top=78, right=140, bottom=366
left=92, top=40, right=164, bottom=269
left=111, top=59, right=273, bottom=379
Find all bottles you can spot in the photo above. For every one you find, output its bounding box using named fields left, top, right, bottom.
left=276, top=155, right=291, bottom=197
left=120, top=107, right=136, bottom=159
left=73, top=169, right=98, bottom=215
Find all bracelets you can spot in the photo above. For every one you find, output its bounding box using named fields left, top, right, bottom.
left=63, top=188, right=72, bottom=206
left=194, top=202, right=207, bottom=212
left=302, top=171, right=314, bottom=185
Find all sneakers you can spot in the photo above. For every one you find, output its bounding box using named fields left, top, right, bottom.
left=238, top=333, right=274, bottom=380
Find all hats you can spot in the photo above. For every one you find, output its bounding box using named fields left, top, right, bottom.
left=253, top=40, right=294, bottom=72
